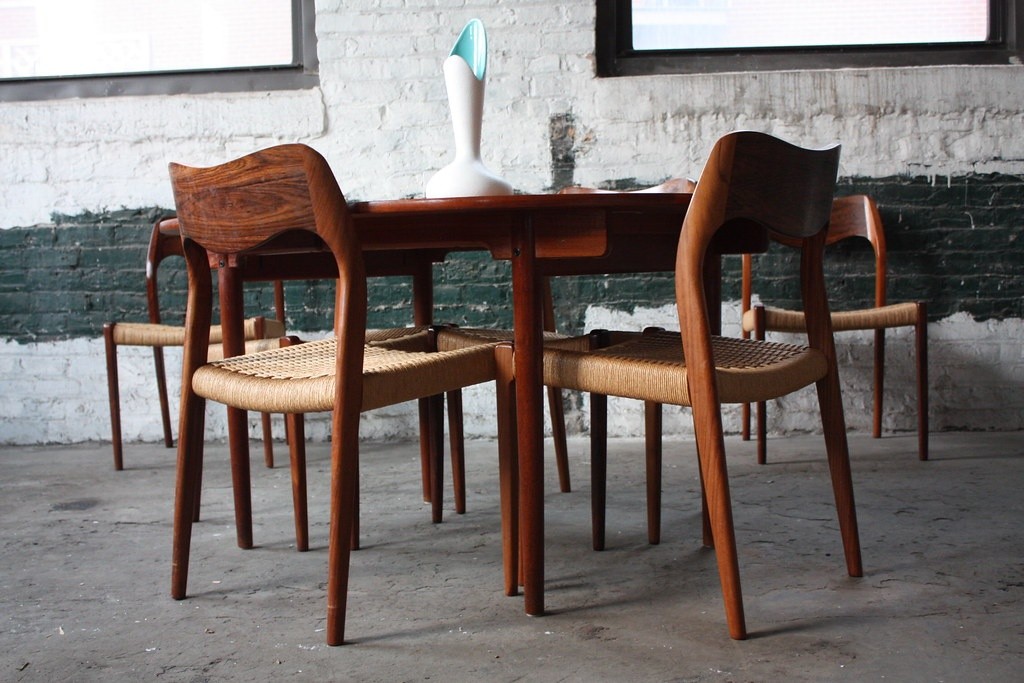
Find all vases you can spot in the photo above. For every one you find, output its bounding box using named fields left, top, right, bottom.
left=425, top=19, right=514, bottom=197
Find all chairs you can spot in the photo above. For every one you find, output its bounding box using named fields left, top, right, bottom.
left=274, top=270, right=442, bottom=551
left=541, top=132, right=864, bottom=641
left=170, top=143, right=519, bottom=646
left=740, top=195, right=931, bottom=463
left=542, top=178, right=698, bottom=492
left=104, top=217, right=286, bottom=473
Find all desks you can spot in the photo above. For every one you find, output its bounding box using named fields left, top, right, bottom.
left=219, top=192, right=723, bottom=616
